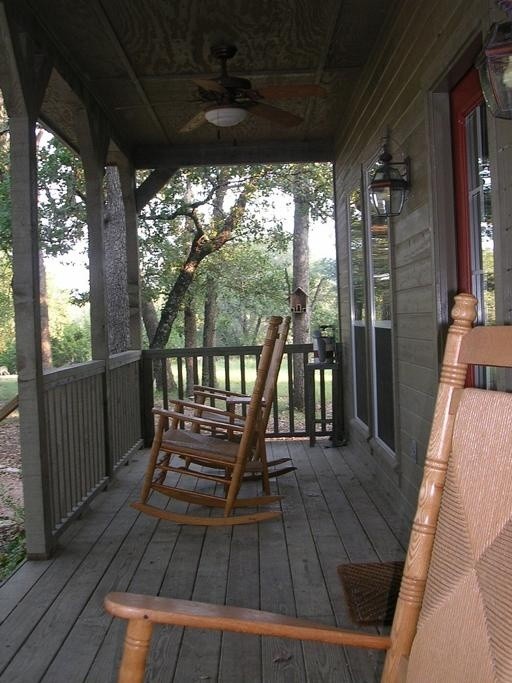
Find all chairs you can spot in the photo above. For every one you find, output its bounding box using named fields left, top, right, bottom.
left=103, top=291, right=512, bottom=682
left=177, top=316, right=298, bottom=483
left=129, top=314, right=285, bottom=527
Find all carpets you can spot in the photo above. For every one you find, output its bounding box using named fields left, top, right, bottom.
left=337, top=559, right=406, bottom=626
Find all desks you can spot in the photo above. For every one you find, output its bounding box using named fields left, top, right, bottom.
left=305, top=363, right=341, bottom=448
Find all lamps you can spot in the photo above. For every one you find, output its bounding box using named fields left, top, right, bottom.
left=367, top=144, right=411, bottom=217
left=474, top=1, right=512, bottom=120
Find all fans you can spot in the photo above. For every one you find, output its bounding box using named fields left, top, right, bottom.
left=177, top=44, right=304, bottom=135
